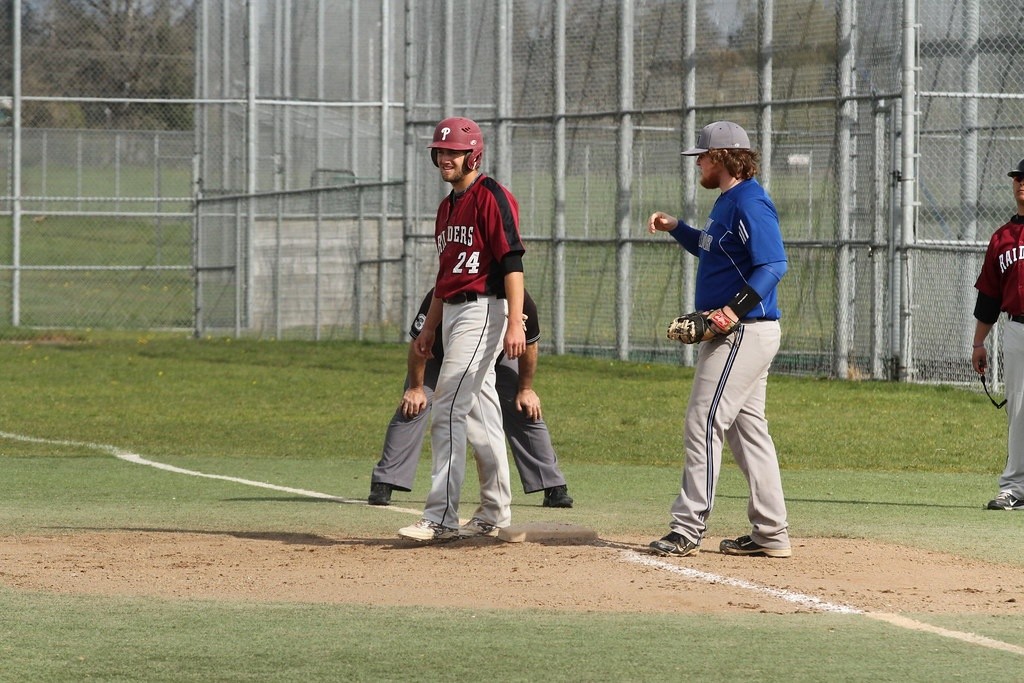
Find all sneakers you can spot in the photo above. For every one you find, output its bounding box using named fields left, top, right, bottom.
left=542, top=485, right=573, bottom=508
left=987, top=492, right=1024, bottom=510
left=457, top=517, right=501, bottom=536
left=368, top=482, right=393, bottom=505
left=398, top=518, right=459, bottom=543
left=648, top=531, right=700, bottom=558
left=719, top=534, right=792, bottom=558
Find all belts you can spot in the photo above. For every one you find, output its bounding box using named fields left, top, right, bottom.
left=441, top=289, right=507, bottom=303
left=740, top=317, right=779, bottom=323
left=1008, top=313, right=1024, bottom=324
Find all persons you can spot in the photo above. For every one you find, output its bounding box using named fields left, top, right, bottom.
left=366, top=284, right=576, bottom=508
left=972, top=158, right=1024, bottom=510
left=645, top=122, right=796, bottom=560
left=396, top=118, right=530, bottom=541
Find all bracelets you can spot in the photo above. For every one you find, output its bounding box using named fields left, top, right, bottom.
left=972, top=344, right=985, bottom=347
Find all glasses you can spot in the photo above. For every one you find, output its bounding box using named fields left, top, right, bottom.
left=1013, top=177, right=1024, bottom=182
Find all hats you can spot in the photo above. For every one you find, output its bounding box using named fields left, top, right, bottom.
left=1007, top=158, right=1024, bottom=177
left=681, top=120, right=751, bottom=155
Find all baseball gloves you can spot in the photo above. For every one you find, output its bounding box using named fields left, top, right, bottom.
left=666, top=309, right=738, bottom=345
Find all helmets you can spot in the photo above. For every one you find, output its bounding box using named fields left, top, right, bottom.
left=427, top=117, right=483, bottom=170
